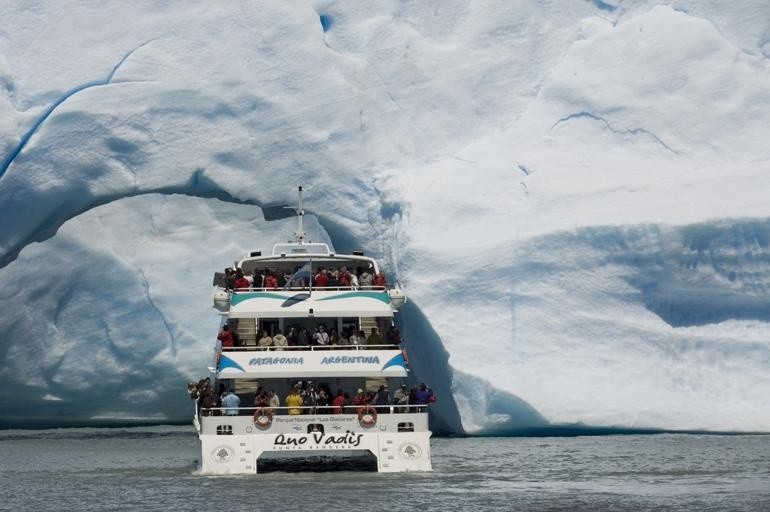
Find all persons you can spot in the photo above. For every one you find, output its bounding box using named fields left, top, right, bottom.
left=224, top=265, right=386, bottom=292
left=186, top=377, right=437, bottom=415
left=257, top=322, right=405, bottom=350
left=217, top=323, right=234, bottom=351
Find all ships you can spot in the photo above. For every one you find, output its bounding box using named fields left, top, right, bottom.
left=186, top=180, right=434, bottom=477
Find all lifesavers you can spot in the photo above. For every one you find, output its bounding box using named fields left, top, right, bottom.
left=358, top=406, right=377, bottom=427
left=254, top=408, right=273, bottom=427
